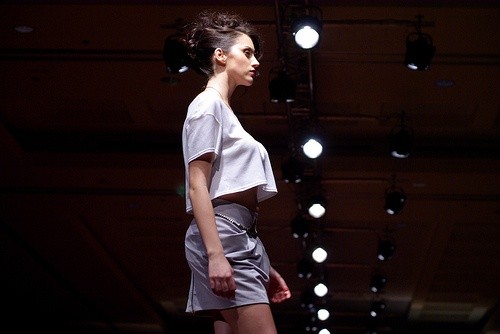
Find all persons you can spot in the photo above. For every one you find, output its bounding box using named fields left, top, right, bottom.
left=162, top=11, right=291, bottom=334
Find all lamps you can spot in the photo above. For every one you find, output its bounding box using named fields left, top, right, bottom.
left=383, top=175, right=407, bottom=215
left=296, top=258, right=315, bottom=280
left=375, top=231, right=398, bottom=262
left=289, top=2, right=323, bottom=49
left=387, top=111, right=416, bottom=161
left=280, top=157, right=304, bottom=185
left=267, top=65, right=297, bottom=104
left=370, top=300, right=387, bottom=318
left=290, top=215, right=309, bottom=239
left=305, top=193, right=328, bottom=219
left=402, top=27, right=441, bottom=73
left=369, top=272, right=389, bottom=294
